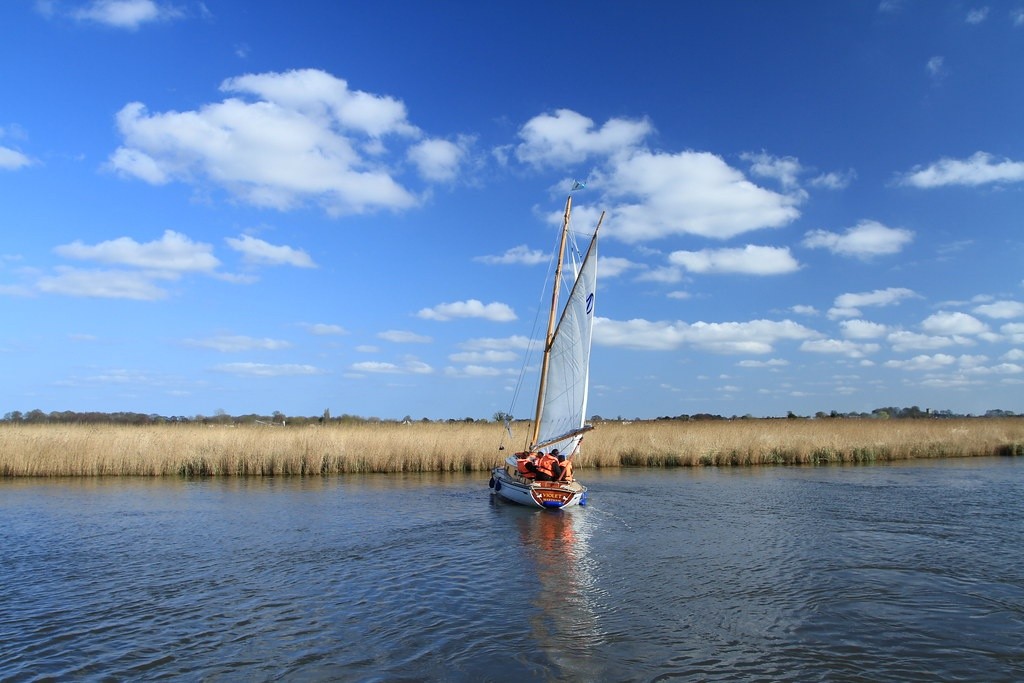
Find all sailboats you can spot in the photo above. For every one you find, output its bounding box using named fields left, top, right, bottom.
left=492, top=197, right=605, bottom=509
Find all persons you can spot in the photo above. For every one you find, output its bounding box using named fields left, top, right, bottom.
left=517, top=450, right=545, bottom=481
left=537, top=449, right=566, bottom=481
left=533, top=452, right=543, bottom=466
left=558, top=455, right=573, bottom=481
